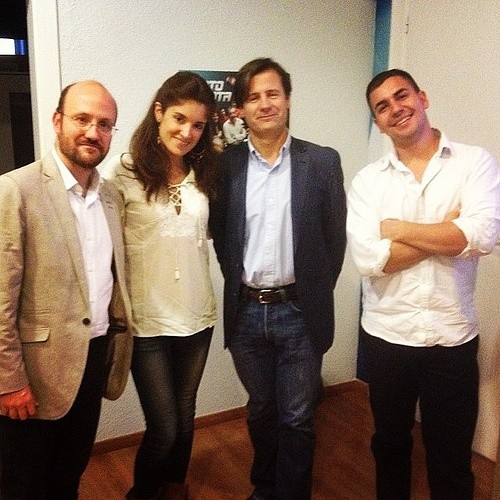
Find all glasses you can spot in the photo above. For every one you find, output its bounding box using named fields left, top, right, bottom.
left=56, top=111, right=119, bottom=138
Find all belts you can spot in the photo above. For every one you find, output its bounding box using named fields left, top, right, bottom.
left=241, top=283, right=296, bottom=305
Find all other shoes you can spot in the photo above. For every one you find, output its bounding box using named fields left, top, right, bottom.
left=159, top=482, right=188, bottom=500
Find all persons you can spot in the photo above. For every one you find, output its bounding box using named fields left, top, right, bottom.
left=0, top=81, right=133, bottom=500
left=102, top=71, right=217, bottom=500
left=208, top=57, right=347, bottom=500
left=346, top=69, right=500, bottom=500
left=210, top=106, right=246, bottom=152
